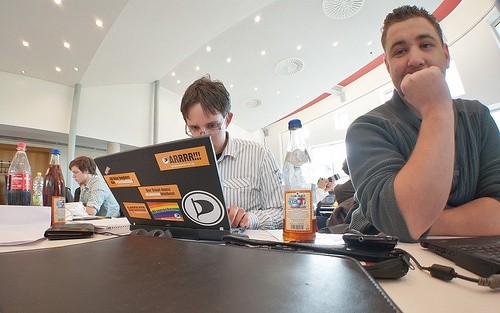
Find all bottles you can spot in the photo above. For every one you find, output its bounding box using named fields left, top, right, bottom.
left=7, top=142, right=32, bottom=205
left=32, top=172, right=44, bottom=206
left=42, top=149, right=66, bottom=228
left=282, top=119, right=316, bottom=244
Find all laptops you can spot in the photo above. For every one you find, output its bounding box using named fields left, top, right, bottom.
left=94, top=135, right=245, bottom=242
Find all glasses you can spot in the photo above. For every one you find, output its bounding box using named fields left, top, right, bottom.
left=185, top=118, right=225, bottom=137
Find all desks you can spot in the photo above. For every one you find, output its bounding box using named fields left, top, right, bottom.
left=0, top=229, right=500, bottom=313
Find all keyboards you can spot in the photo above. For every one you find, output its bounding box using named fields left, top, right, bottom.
left=420, top=235, right=500, bottom=278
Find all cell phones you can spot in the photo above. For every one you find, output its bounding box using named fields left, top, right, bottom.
left=343, top=234, right=398, bottom=251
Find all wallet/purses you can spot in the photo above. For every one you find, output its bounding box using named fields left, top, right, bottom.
left=44, top=222, right=95, bottom=240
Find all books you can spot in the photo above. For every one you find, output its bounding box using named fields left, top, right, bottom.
left=71, top=218, right=131, bottom=233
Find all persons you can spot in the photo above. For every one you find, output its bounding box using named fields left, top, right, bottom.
left=180, top=73, right=284, bottom=230
left=345, top=4, right=500, bottom=243
left=316, top=159, right=357, bottom=216
left=69, top=156, right=120, bottom=217
